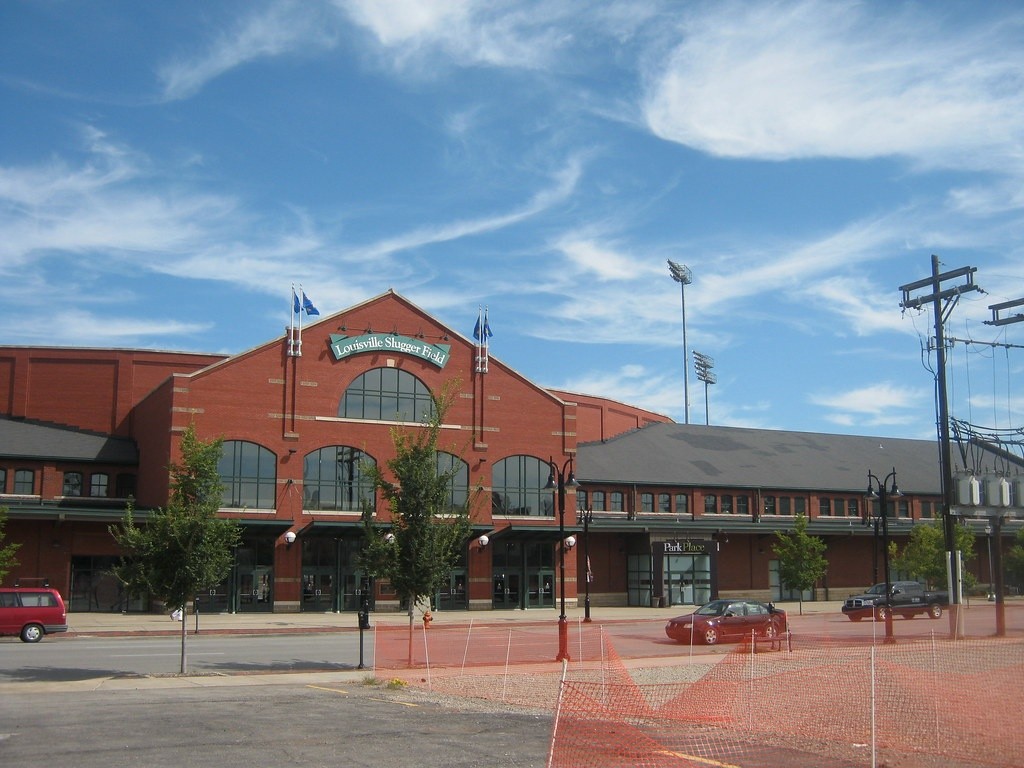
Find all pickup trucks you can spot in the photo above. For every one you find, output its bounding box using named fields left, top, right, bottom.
left=841, top=580, right=956, bottom=622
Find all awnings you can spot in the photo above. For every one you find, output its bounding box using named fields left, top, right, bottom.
left=297, top=520, right=398, bottom=539
left=490, top=525, right=583, bottom=542
left=426, top=524, right=494, bottom=544
left=207, top=516, right=294, bottom=541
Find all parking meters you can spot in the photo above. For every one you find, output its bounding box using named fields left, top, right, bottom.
left=766, top=601, right=776, bottom=651
left=357, top=607, right=371, bottom=670
left=193, top=595, right=201, bottom=634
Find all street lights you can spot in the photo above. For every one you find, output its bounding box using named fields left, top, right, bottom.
left=984, top=524, right=995, bottom=602
left=692, top=349, right=717, bottom=425
left=542, top=450, right=583, bottom=664
left=357, top=499, right=378, bottom=631
left=666, top=258, right=695, bottom=425
left=866, top=510, right=882, bottom=593
left=576, top=503, right=595, bottom=624
left=861, top=466, right=905, bottom=644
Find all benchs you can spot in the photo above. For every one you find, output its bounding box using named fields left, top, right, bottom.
left=742, top=629, right=796, bottom=654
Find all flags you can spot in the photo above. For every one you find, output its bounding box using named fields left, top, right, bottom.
left=293, top=291, right=304, bottom=313
left=301, top=292, right=319, bottom=315
left=473, top=315, right=483, bottom=344
left=483, top=314, right=493, bottom=342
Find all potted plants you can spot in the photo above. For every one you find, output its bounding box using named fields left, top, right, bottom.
left=649, top=570, right=663, bottom=608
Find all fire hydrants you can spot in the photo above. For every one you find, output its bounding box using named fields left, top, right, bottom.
left=422, top=611, right=434, bottom=629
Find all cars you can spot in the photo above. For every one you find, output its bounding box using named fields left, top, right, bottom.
left=664, top=598, right=788, bottom=647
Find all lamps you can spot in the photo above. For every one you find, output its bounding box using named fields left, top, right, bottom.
left=478, top=534, right=489, bottom=551
left=386, top=533, right=395, bottom=547
left=565, top=536, right=576, bottom=551
left=284, top=531, right=297, bottom=551
left=390, top=326, right=399, bottom=336
left=441, top=329, right=449, bottom=342
left=365, top=321, right=373, bottom=334
left=340, top=320, right=347, bottom=331
left=416, top=327, right=425, bottom=339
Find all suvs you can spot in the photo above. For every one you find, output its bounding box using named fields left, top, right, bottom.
left=0, top=582, right=70, bottom=643
